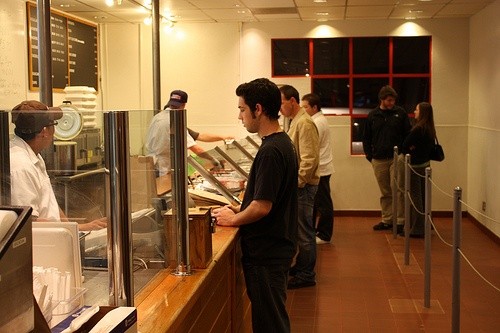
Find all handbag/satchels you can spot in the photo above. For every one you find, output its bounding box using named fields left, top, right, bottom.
left=430, top=136, right=444, bottom=161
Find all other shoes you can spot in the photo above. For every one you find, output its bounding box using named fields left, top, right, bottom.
left=315, top=235, right=331, bottom=244
left=395, top=225, right=403, bottom=233
left=401, top=233, right=422, bottom=238
left=373, top=222, right=392, bottom=230
left=288, top=276, right=315, bottom=289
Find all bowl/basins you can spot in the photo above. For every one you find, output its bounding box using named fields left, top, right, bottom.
left=64, top=86, right=97, bottom=128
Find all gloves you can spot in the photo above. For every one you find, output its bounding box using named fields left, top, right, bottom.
left=211, top=157, right=221, bottom=171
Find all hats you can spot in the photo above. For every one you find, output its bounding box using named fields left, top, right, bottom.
left=169, top=90, right=188, bottom=107
left=11, top=100, right=63, bottom=133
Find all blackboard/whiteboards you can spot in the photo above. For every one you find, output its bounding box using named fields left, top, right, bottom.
left=27, top=2, right=98, bottom=93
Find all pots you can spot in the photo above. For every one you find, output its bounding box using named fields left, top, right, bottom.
left=54, top=141, right=78, bottom=176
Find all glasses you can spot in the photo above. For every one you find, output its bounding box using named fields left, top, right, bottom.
left=46, top=120, right=58, bottom=126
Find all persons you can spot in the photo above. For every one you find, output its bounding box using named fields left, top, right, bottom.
left=304, top=93, right=335, bottom=242
left=10, top=100, right=108, bottom=230
left=144, top=97, right=222, bottom=180
left=407, top=102, right=434, bottom=237
left=171, top=90, right=236, bottom=144
left=281, top=85, right=319, bottom=288
left=361, top=87, right=410, bottom=237
left=212, top=78, right=300, bottom=333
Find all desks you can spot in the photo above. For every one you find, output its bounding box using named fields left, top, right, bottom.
left=53, top=164, right=106, bottom=218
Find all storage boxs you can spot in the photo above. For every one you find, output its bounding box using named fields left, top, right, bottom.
left=163, top=208, right=213, bottom=269
left=70, top=305, right=137, bottom=333
